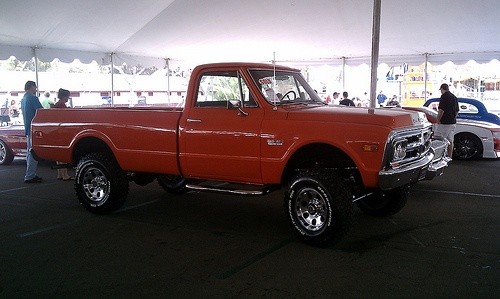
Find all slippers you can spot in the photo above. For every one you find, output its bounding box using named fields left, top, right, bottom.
left=57, top=177, right=63, bottom=180
left=62, top=177, right=74, bottom=180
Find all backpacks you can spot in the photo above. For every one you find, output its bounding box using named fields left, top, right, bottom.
left=12, top=106, right=20, bottom=117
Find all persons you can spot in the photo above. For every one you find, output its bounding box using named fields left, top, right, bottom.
left=10, top=101, right=20, bottom=126
left=435, top=83, right=459, bottom=160
left=314, top=91, right=401, bottom=108
left=52, top=90, right=74, bottom=180
left=22, top=82, right=43, bottom=183
left=135, top=91, right=148, bottom=104
left=38, top=91, right=54, bottom=108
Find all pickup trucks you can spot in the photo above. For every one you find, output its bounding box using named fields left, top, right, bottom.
left=29, top=62, right=453, bottom=248
left=0, top=125, right=28, bottom=165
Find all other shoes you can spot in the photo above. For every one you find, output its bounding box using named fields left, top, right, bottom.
left=25, top=176, right=42, bottom=184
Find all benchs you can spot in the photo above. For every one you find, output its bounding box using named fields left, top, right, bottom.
left=195, top=101, right=255, bottom=107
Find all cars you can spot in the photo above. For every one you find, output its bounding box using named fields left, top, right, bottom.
left=377, top=105, right=500, bottom=162
left=421, top=97, right=500, bottom=127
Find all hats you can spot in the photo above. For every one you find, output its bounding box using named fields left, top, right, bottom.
left=439, top=83, right=449, bottom=90
